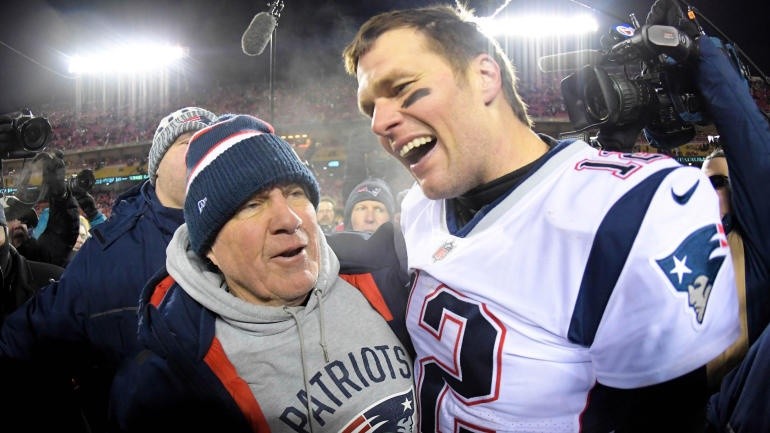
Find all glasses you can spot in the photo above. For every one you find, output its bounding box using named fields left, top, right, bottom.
left=709, top=175, right=730, bottom=189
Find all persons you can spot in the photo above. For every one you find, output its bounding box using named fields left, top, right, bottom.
left=343, top=4, right=750, bottom=433
left=0, top=107, right=410, bottom=433
left=645, top=0, right=770, bottom=433
left=108, top=113, right=419, bottom=433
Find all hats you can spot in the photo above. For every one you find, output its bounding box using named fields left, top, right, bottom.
left=148, top=107, right=218, bottom=185
left=184, top=115, right=320, bottom=257
left=0, top=196, right=39, bottom=227
left=343, top=178, right=396, bottom=224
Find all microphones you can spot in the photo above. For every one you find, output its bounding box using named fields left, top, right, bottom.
left=241, top=12, right=276, bottom=56
left=538, top=49, right=605, bottom=72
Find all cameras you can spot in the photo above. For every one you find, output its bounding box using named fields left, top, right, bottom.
left=64, top=168, right=95, bottom=197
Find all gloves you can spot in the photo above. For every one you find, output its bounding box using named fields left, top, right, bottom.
left=0, top=115, right=15, bottom=151
left=646, top=0, right=700, bottom=68
left=42, top=150, right=66, bottom=198
left=77, top=193, right=98, bottom=217
